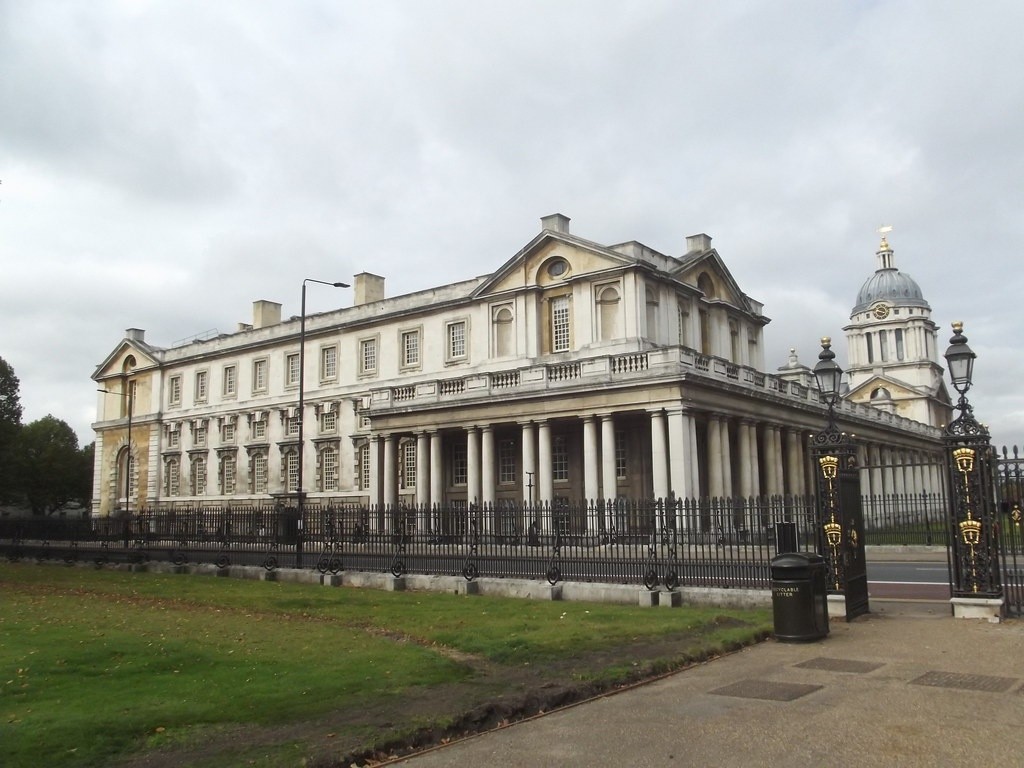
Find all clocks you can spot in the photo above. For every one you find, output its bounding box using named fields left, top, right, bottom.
left=872, top=304, right=890, bottom=321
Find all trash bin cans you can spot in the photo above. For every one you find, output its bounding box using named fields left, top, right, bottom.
left=276, top=507, right=298, bottom=546
left=770, top=552, right=830, bottom=643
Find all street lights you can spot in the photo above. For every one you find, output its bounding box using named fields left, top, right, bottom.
left=96, top=387, right=134, bottom=543
left=297, top=278, right=350, bottom=530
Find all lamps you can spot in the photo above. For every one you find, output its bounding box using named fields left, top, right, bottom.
left=944, top=320, right=977, bottom=396
left=813, top=337, right=844, bottom=409
